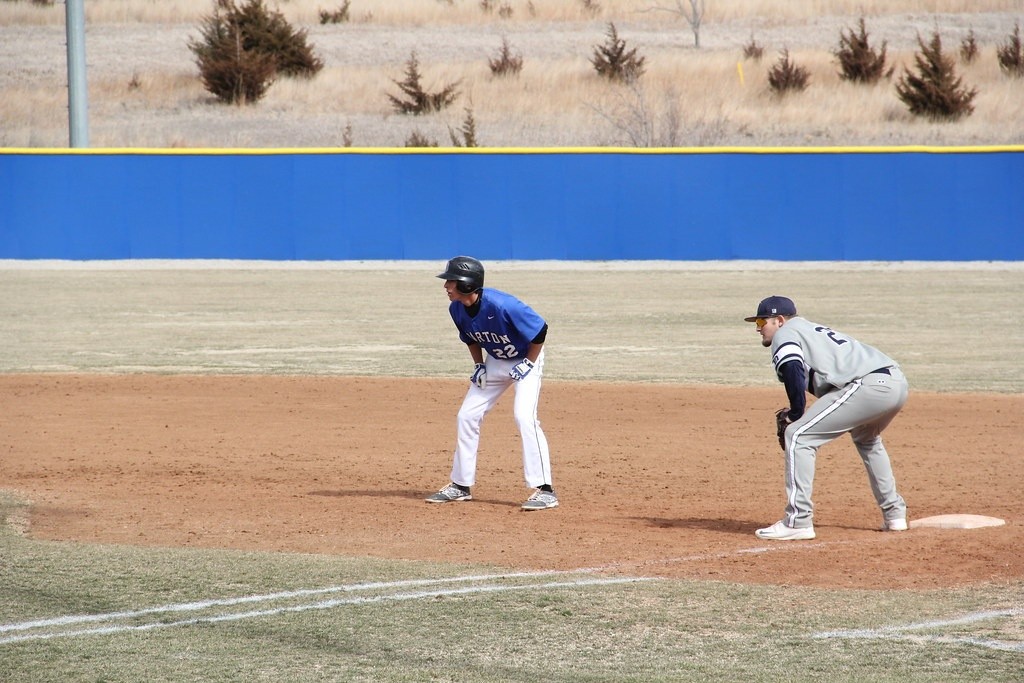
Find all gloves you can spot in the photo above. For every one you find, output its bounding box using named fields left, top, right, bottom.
left=469, top=363, right=487, bottom=391
left=509, top=358, right=534, bottom=382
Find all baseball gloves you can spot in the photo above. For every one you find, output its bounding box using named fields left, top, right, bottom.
left=774, top=407, right=791, bottom=451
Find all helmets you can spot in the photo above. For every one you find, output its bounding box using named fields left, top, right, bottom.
left=435, top=255, right=485, bottom=294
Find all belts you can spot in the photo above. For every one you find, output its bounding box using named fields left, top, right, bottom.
left=870, top=368, right=891, bottom=376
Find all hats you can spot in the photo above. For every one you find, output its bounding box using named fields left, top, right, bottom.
left=744, top=295, right=796, bottom=322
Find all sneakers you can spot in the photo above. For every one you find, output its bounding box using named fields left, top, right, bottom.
left=425, top=483, right=473, bottom=503
left=520, top=487, right=559, bottom=510
left=879, top=519, right=909, bottom=532
left=755, top=520, right=816, bottom=540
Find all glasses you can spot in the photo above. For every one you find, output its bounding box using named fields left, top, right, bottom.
left=755, top=318, right=767, bottom=327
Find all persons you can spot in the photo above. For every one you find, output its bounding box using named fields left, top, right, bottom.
left=744, top=295, right=909, bottom=539
left=425, top=256, right=558, bottom=510
